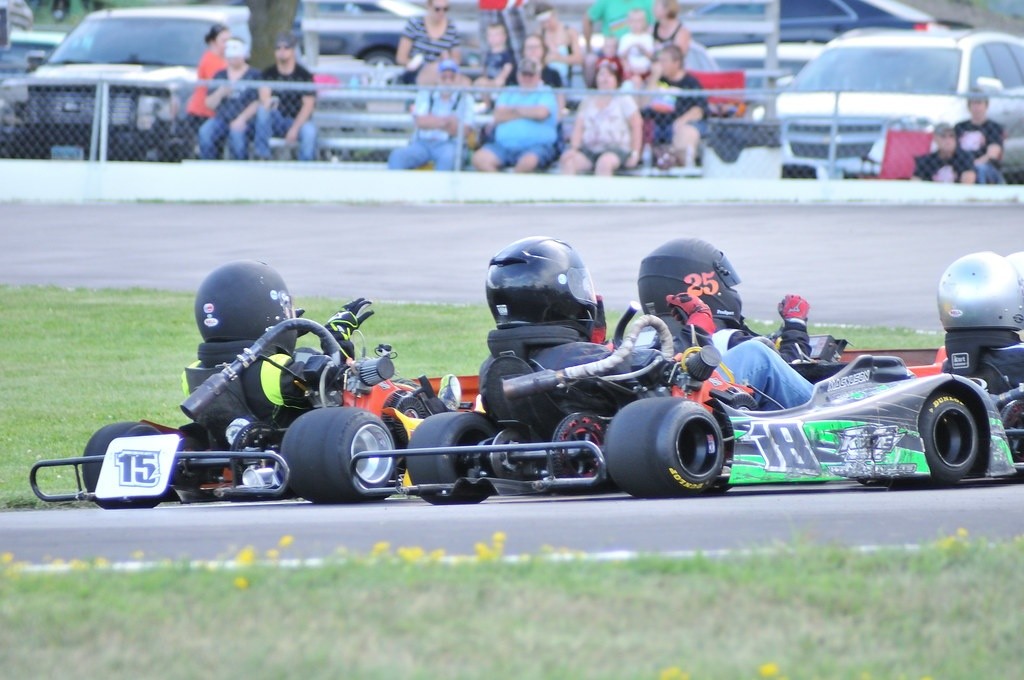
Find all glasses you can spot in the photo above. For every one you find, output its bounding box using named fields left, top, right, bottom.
left=522, top=71, right=534, bottom=76
left=275, top=45, right=292, bottom=49
left=433, top=7, right=448, bottom=13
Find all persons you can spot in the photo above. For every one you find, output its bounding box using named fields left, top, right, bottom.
left=471, top=59, right=561, bottom=173
left=198, top=38, right=260, bottom=160
left=181, top=259, right=461, bottom=452
left=911, top=123, right=976, bottom=185
left=560, top=66, right=643, bottom=176
left=474, top=235, right=814, bottom=413
left=185, top=27, right=230, bottom=133
left=254, top=37, right=317, bottom=161
left=953, top=89, right=1003, bottom=184
left=390, top=0, right=690, bottom=144
left=638, top=238, right=812, bottom=362
left=388, top=59, right=476, bottom=171
left=938, top=251, right=1024, bottom=388
left=640, top=46, right=707, bottom=167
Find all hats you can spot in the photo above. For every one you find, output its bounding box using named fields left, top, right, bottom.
left=437, top=61, right=458, bottom=74
left=223, top=40, right=244, bottom=58
left=519, top=58, right=535, bottom=74
left=277, top=30, right=295, bottom=45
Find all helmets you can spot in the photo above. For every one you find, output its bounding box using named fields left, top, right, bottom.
left=937, top=251, right=1024, bottom=330
left=195, top=259, right=296, bottom=356
left=638, top=237, right=742, bottom=330
left=486, top=236, right=597, bottom=342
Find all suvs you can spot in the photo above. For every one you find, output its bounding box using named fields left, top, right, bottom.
left=753, top=29, right=1023, bottom=183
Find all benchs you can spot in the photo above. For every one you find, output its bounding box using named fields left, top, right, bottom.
left=184, top=88, right=779, bottom=176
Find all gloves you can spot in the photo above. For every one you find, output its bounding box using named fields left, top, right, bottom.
left=590, top=293, right=607, bottom=343
left=778, top=293, right=809, bottom=324
left=323, top=298, right=374, bottom=341
left=667, top=293, right=713, bottom=336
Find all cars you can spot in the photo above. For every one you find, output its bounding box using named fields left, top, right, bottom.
left=230, top=0, right=430, bottom=86
left=0, top=7, right=255, bottom=159
left=706, top=37, right=824, bottom=100
left=669, top=1, right=938, bottom=45
